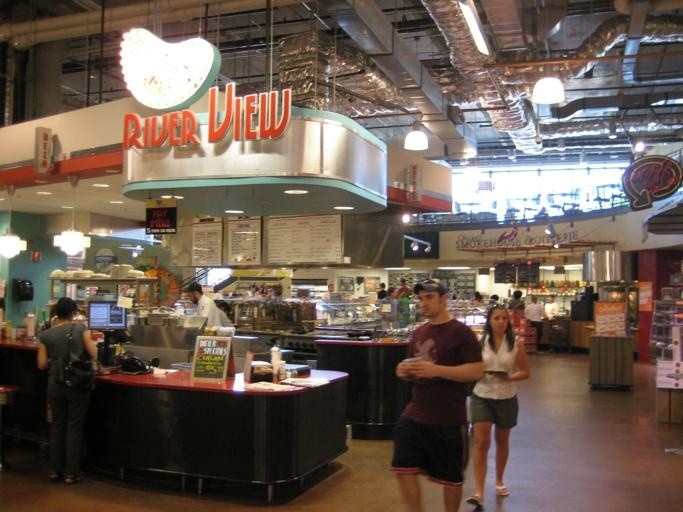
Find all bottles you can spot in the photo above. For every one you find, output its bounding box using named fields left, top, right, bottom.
left=538, top=279, right=583, bottom=287
left=3, top=321, right=12, bottom=339
left=26, top=313, right=35, bottom=336
left=278, top=360, right=288, bottom=382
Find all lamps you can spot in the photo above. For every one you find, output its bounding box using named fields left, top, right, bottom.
left=116, top=2, right=221, bottom=111
left=530, top=67, right=566, bottom=107
left=51, top=174, right=91, bottom=258
left=1, top=185, right=28, bottom=260
left=403, top=114, right=431, bottom=150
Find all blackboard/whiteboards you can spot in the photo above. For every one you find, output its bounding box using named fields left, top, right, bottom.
left=191, top=335, right=232, bottom=384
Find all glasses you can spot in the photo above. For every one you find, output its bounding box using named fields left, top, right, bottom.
left=492, top=304, right=506, bottom=309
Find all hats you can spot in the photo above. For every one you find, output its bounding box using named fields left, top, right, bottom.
left=413, top=279, right=446, bottom=294
left=184, top=282, right=202, bottom=291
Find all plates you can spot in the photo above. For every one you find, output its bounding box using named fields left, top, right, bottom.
left=109, top=264, right=132, bottom=279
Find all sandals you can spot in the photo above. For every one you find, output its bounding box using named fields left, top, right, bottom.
left=46, top=469, right=61, bottom=481
left=63, top=471, right=87, bottom=484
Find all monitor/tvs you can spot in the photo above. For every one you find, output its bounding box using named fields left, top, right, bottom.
left=88, top=300, right=127, bottom=331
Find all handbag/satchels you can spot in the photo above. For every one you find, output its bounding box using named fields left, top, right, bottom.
left=63, top=361, right=95, bottom=393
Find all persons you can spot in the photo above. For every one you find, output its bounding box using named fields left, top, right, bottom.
left=181, top=280, right=222, bottom=334
left=471, top=290, right=559, bottom=354
left=376, top=283, right=387, bottom=299
left=35, top=296, right=98, bottom=485
left=464, top=303, right=530, bottom=509
left=395, top=277, right=410, bottom=298
left=323, top=283, right=334, bottom=300
left=379, top=287, right=400, bottom=322
left=387, top=279, right=485, bottom=512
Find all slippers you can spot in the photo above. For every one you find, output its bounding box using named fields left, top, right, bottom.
left=495, top=484, right=509, bottom=497
left=466, top=494, right=485, bottom=507
